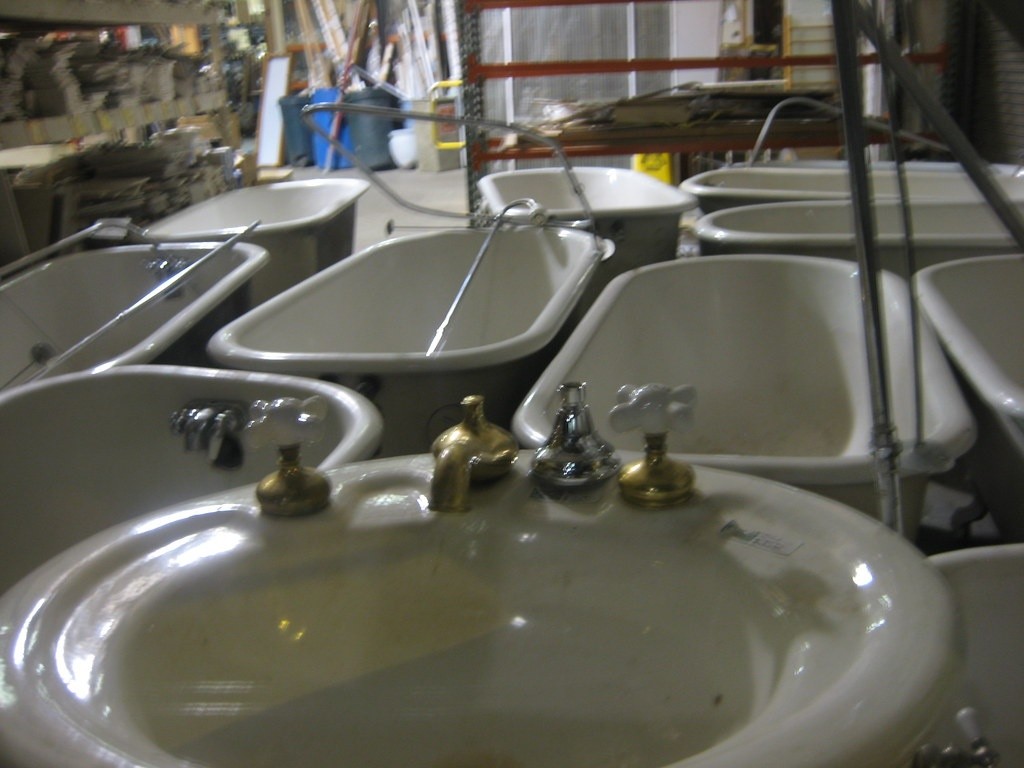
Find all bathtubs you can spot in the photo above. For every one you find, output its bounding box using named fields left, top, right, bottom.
left=0, top=356, right=384, bottom=595
left=0, top=156, right=1024, bottom=492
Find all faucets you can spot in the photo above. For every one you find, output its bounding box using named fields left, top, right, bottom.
left=178, top=407, right=216, bottom=453
left=424, top=393, right=521, bottom=517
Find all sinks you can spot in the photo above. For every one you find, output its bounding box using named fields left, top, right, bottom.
left=0, top=446, right=958, bottom=768
